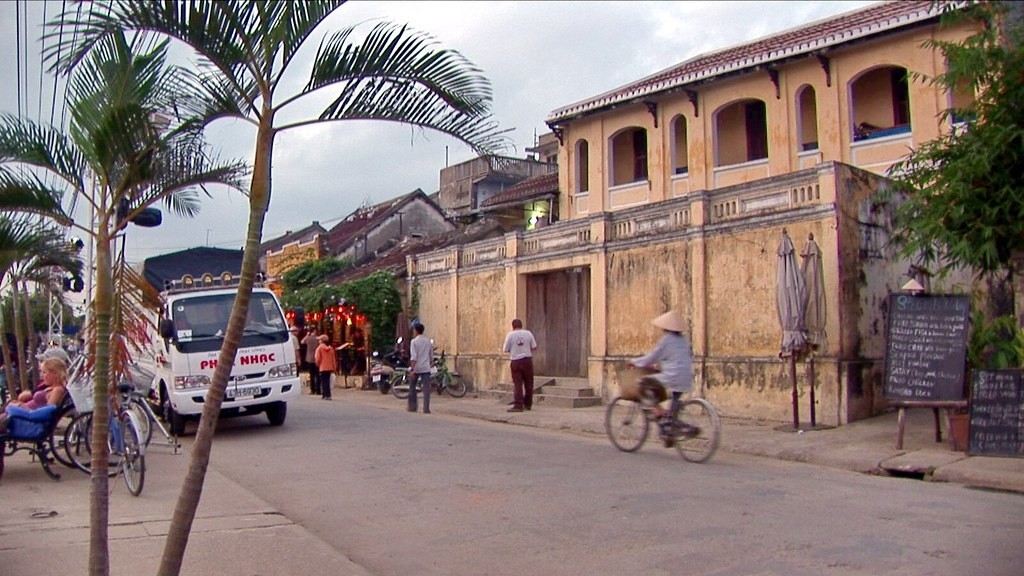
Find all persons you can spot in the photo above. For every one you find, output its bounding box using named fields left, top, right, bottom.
left=0, top=329, right=85, bottom=442
left=627, top=312, right=694, bottom=447
left=503, top=319, right=537, bottom=412
left=290, top=325, right=365, bottom=400
left=406, top=323, right=433, bottom=414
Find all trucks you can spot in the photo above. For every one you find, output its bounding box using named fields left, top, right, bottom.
left=121, top=246, right=305, bottom=437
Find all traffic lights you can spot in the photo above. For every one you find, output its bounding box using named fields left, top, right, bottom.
left=63, top=236, right=84, bottom=292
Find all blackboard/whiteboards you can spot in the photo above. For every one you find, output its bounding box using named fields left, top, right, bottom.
left=967, top=368, right=1024, bottom=459
left=881, top=293, right=969, bottom=407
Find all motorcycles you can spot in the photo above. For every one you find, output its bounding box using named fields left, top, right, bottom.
left=370, top=336, right=465, bottom=399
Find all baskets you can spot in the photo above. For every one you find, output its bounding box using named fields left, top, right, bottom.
left=66, top=375, right=94, bottom=413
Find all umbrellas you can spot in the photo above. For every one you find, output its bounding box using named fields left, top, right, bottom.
left=776, top=228, right=829, bottom=427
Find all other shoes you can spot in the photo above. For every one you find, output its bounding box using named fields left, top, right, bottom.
left=507, top=407, right=524, bottom=412
left=309, top=392, right=332, bottom=400
left=525, top=406, right=531, bottom=409
left=423, top=410, right=431, bottom=414
left=406, top=408, right=416, bottom=412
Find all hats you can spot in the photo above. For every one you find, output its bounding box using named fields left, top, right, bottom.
left=650, top=310, right=689, bottom=332
left=34, top=347, right=67, bottom=363
left=315, top=334, right=329, bottom=342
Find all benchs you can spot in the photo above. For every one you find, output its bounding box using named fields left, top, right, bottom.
left=0, top=389, right=74, bottom=482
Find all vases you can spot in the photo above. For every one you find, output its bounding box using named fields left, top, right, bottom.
left=948, top=411, right=971, bottom=450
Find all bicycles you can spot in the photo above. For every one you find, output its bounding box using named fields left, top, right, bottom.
left=604, top=364, right=719, bottom=464
left=49, top=361, right=155, bottom=497
